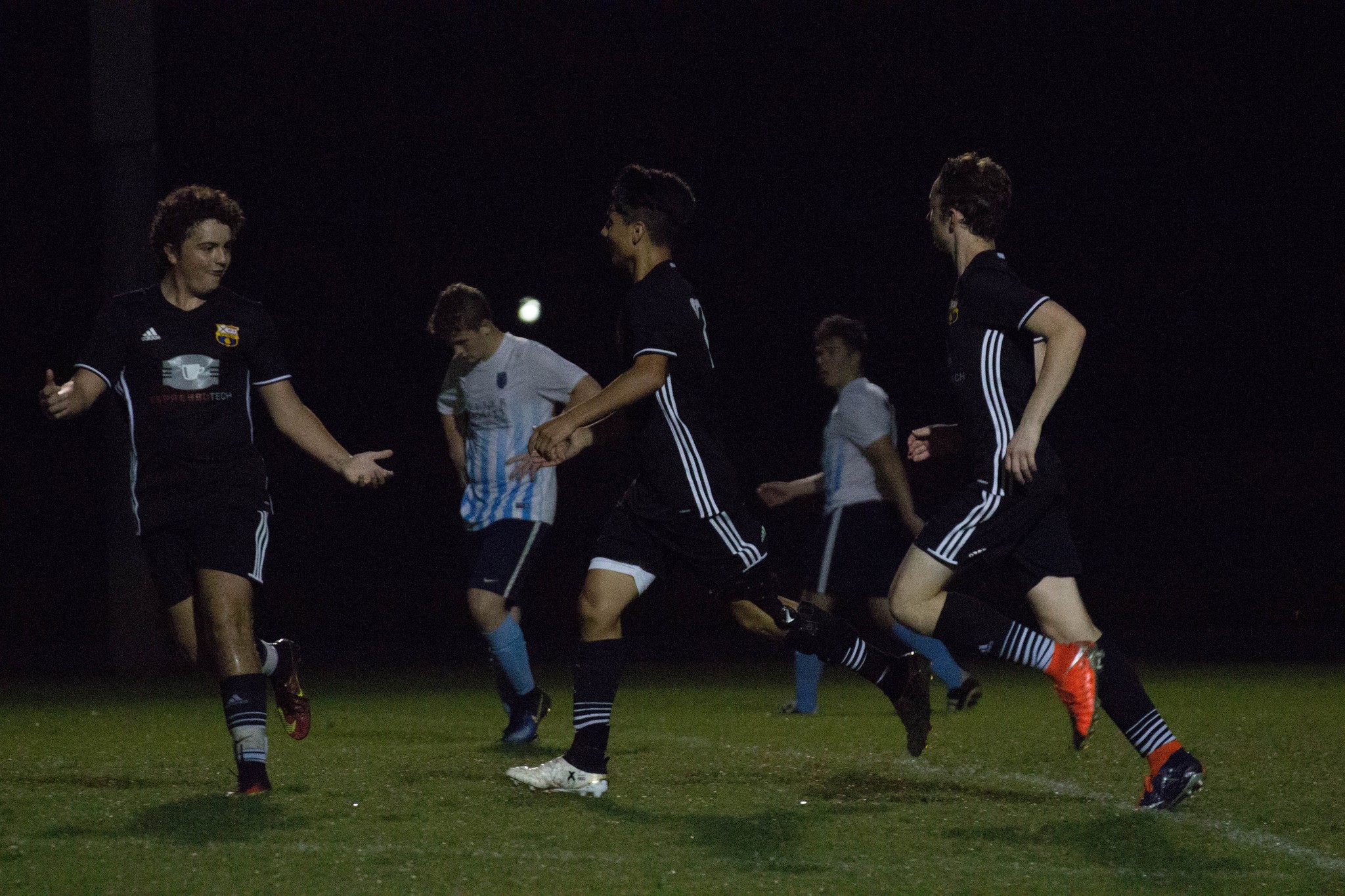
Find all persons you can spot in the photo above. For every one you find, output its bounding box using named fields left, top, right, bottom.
left=755, top=316, right=982, bottom=718
left=886, top=151, right=1205, bottom=813
left=38, top=183, right=395, bottom=800
left=430, top=283, right=606, bottom=752
left=504, top=167, right=936, bottom=799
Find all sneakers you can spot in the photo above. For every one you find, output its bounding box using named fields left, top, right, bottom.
left=779, top=704, right=801, bottom=716
left=946, top=670, right=982, bottom=713
left=891, top=651, right=934, bottom=757
left=502, top=685, right=550, bottom=742
left=268, top=638, right=311, bottom=740
left=227, top=744, right=271, bottom=798
left=1133, top=747, right=1205, bottom=812
left=505, top=755, right=609, bottom=799
left=1053, top=640, right=1104, bottom=752
